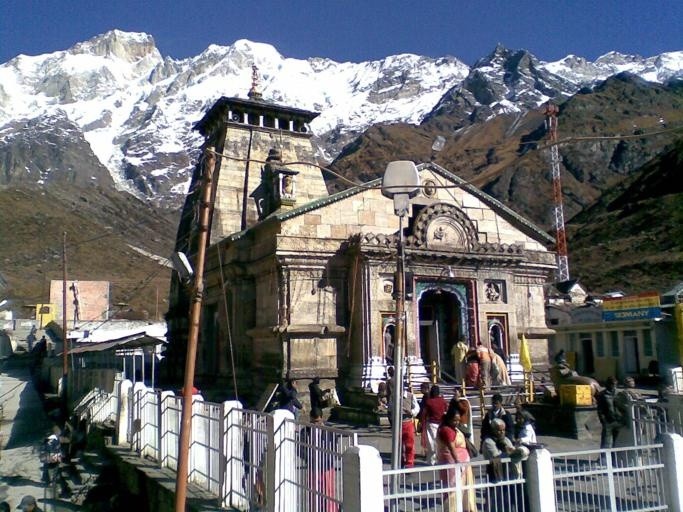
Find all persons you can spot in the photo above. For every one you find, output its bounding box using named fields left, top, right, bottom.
left=554, top=349, right=568, bottom=364
left=584, top=292, right=595, bottom=306
left=16, top=495, right=43, bottom=512
left=0, top=501, right=10, bottom=512
left=279, top=378, right=305, bottom=416
left=620, top=375, right=643, bottom=403
left=596, top=373, right=622, bottom=468
left=375, top=337, right=538, bottom=512
left=62, top=420, right=74, bottom=455
left=308, top=377, right=332, bottom=425
left=49, top=420, right=62, bottom=436
